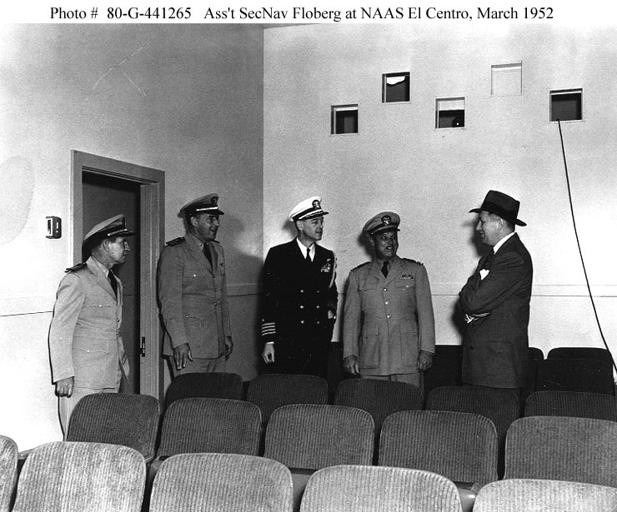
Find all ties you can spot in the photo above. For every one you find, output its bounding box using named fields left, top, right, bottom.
left=306, top=248, right=312, bottom=264
left=109, top=270, right=119, bottom=301
left=380, top=261, right=390, bottom=279
left=202, top=243, right=214, bottom=269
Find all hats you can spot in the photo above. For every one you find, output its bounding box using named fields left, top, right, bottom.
left=179, top=193, right=225, bottom=218
left=287, top=195, right=329, bottom=222
left=469, top=190, right=528, bottom=227
left=362, top=211, right=401, bottom=236
left=83, top=213, right=136, bottom=242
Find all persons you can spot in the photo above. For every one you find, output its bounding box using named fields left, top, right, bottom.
left=458, top=191, right=534, bottom=389
left=343, top=212, right=436, bottom=392
left=49, top=213, right=131, bottom=442
left=256, top=195, right=337, bottom=377
left=158, top=193, right=234, bottom=379
